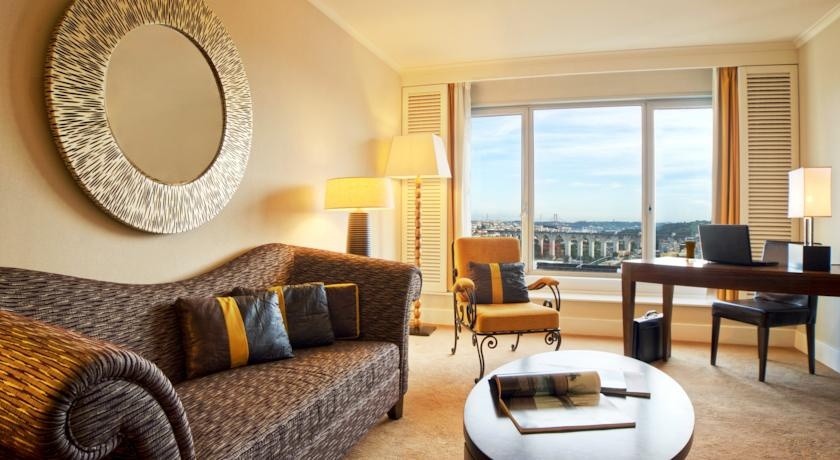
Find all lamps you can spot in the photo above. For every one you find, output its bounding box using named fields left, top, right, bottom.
left=788, top=167, right=831, bottom=272
left=385, top=134, right=452, bottom=336
left=324, top=176, right=394, bottom=258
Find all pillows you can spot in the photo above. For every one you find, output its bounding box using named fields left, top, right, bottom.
left=176, top=292, right=295, bottom=381
left=467, top=260, right=530, bottom=304
left=324, top=283, right=360, bottom=340
left=233, top=281, right=334, bottom=348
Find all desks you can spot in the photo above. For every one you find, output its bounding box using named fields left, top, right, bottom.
left=621, top=256, right=840, bottom=362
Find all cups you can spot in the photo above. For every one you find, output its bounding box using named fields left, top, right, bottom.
left=686, top=240, right=695, bottom=258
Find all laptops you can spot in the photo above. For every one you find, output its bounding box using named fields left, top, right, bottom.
left=699, top=222, right=780, bottom=266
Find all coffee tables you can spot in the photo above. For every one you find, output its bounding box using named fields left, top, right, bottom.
left=462, top=349, right=695, bottom=460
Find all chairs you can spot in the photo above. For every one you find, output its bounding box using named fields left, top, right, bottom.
left=451, top=237, right=561, bottom=384
left=711, top=240, right=822, bottom=382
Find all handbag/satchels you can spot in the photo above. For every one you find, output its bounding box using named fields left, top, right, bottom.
left=631, top=310, right=665, bottom=363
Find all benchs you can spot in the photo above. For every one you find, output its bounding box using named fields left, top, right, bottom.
left=0, top=243, right=423, bottom=460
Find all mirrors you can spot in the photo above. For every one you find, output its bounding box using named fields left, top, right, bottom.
left=44, top=0, right=254, bottom=234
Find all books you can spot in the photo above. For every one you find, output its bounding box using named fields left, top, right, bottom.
left=597, top=365, right=651, bottom=399
left=488, top=370, right=636, bottom=434
